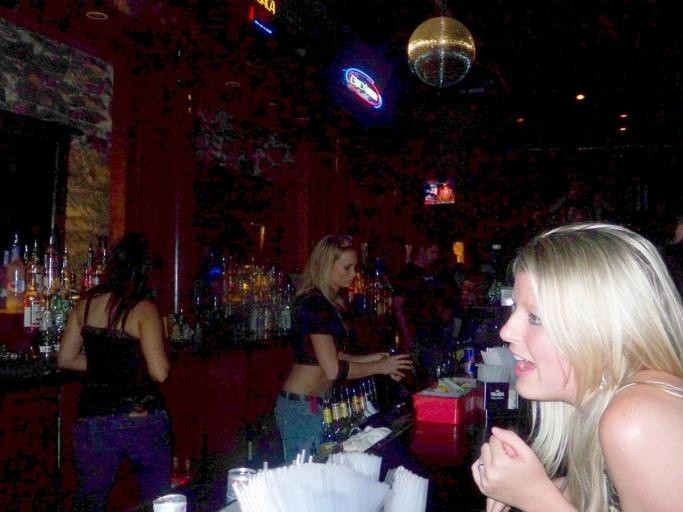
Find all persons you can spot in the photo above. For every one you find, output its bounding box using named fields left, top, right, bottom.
left=54, top=230, right=176, bottom=512
left=393, top=238, right=440, bottom=348
left=271, top=233, right=415, bottom=466
left=461, top=220, right=683, bottom=512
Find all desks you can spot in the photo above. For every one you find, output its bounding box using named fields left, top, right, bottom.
left=215, top=382, right=531, bottom=512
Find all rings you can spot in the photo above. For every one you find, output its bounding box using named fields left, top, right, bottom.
left=477, top=463, right=485, bottom=470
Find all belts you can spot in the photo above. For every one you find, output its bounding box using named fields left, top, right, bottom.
left=277, top=387, right=325, bottom=405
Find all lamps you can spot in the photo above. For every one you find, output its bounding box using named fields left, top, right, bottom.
left=406, top=0, right=477, bottom=87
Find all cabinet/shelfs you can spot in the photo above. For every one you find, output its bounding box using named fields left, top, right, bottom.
left=0, top=320, right=294, bottom=512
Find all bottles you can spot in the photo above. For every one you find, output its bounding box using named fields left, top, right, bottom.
left=170, top=457, right=195, bottom=488
left=487, top=279, right=502, bottom=305
left=162, top=309, right=206, bottom=362
left=322, top=376, right=382, bottom=438
left=345, top=243, right=394, bottom=318
left=206, top=253, right=296, bottom=345
left=193, top=280, right=203, bottom=309
left=429, top=337, right=477, bottom=389
left=0, top=232, right=109, bottom=375
